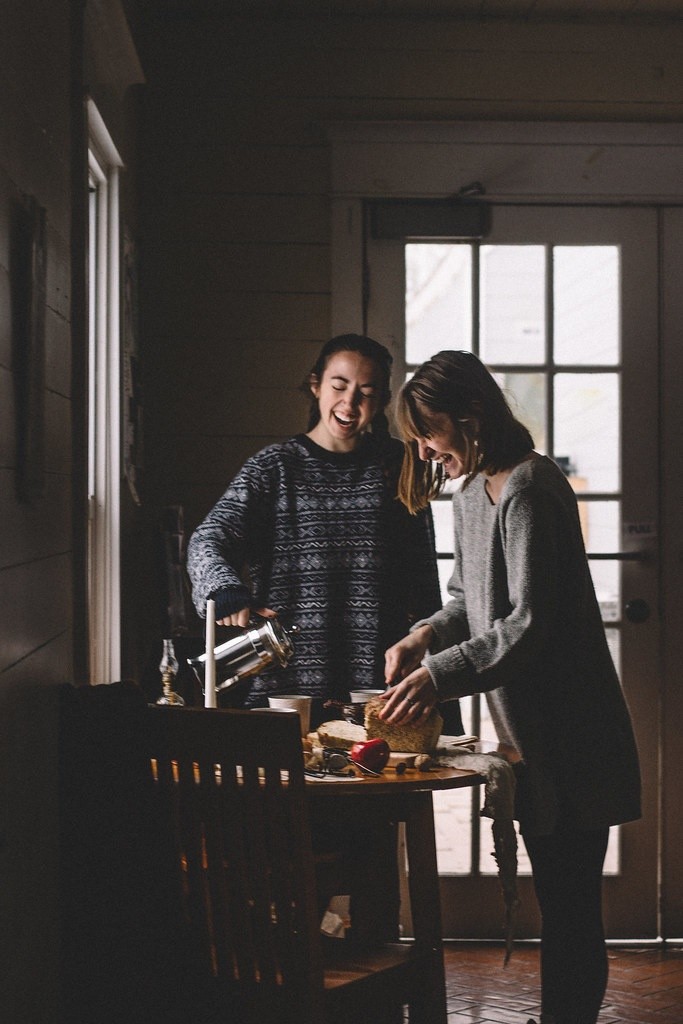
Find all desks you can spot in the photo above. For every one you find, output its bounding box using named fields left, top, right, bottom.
left=151, top=741, right=523, bottom=1024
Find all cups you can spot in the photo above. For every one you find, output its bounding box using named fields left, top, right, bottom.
left=350, top=689, right=385, bottom=703
left=267, top=695, right=312, bottom=738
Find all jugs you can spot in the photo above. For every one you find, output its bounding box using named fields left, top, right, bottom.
left=186, top=612, right=301, bottom=701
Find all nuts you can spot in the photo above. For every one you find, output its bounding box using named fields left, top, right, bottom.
left=414, top=755, right=432, bottom=771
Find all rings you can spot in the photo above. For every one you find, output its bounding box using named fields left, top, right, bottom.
left=404, top=696, right=414, bottom=705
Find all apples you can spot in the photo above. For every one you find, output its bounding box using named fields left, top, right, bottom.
left=351, top=737, right=390, bottom=773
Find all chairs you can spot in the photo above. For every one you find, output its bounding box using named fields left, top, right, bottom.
left=58, top=678, right=418, bottom=1024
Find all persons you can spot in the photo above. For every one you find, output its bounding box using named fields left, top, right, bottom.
left=377, top=349, right=644, bottom=1024
left=185, top=334, right=468, bottom=942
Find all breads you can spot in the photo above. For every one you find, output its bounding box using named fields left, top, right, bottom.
left=316, top=720, right=368, bottom=749
left=364, top=696, right=445, bottom=753
